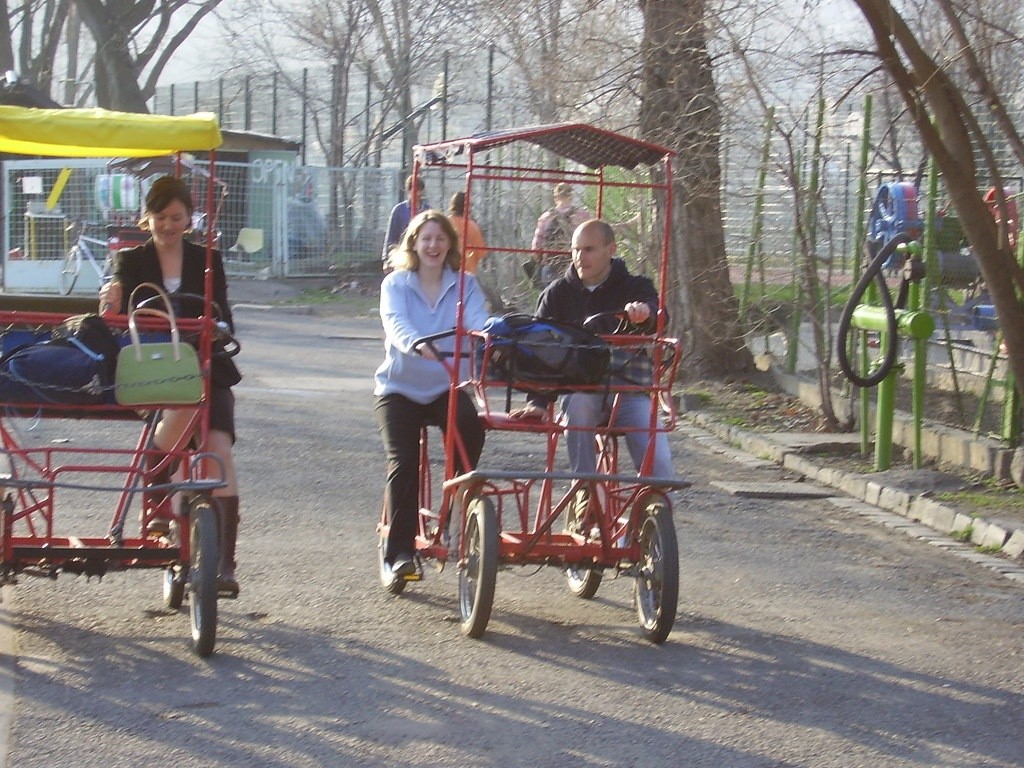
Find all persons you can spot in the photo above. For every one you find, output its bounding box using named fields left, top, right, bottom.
left=374, top=210, right=488, bottom=576
left=448, top=191, right=487, bottom=275
left=531, top=182, right=594, bottom=289
left=381, top=175, right=432, bottom=275
left=505, top=220, right=674, bottom=613
left=99, top=176, right=239, bottom=598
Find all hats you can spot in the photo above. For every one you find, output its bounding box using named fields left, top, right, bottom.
left=553, top=181, right=575, bottom=196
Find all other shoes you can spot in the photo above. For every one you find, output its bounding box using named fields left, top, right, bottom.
left=392, top=552, right=416, bottom=574
left=439, top=524, right=449, bottom=546
left=574, top=487, right=589, bottom=522
left=619, top=557, right=635, bottom=568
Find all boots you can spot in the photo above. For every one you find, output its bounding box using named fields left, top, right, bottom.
left=210, top=496, right=239, bottom=596
left=146, top=440, right=171, bottom=535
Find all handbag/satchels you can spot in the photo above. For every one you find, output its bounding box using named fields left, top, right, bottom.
left=136, top=292, right=240, bottom=361
left=522, top=255, right=543, bottom=290
left=114, top=282, right=206, bottom=406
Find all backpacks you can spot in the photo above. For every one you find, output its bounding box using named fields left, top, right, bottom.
left=0, top=336, right=106, bottom=404
left=476, top=312, right=611, bottom=402
left=542, top=203, right=589, bottom=276
left=58, top=312, right=120, bottom=385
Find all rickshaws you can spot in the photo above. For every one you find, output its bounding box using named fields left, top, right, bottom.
left=377, top=120, right=694, bottom=645
left=0, top=103, right=241, bottom=661
